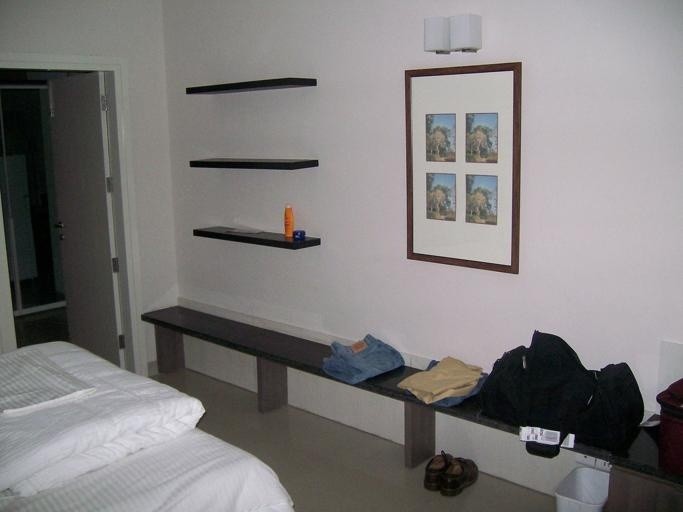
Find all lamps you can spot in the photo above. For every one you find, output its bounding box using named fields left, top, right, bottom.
left=423, top=14, right=482, bottom=56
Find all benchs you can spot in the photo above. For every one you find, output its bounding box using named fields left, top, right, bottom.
left=139, top=305, right=613, bottom=468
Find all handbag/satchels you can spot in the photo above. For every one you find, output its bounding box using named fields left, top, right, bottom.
left=478, top=330, right=643, bottom=456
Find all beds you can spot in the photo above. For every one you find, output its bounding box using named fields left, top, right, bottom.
left=1, top=339, right=295, bottom=511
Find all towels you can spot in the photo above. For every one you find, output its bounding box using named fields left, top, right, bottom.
left=2, top=348, right=97, bottom=418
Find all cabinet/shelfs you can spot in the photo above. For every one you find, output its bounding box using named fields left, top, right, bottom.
left=186, top=77, right=320, bottom=251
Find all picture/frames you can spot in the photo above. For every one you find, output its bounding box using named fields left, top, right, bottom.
left=405, top=61, right=524, bottom=274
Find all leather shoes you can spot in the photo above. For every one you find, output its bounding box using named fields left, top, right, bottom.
left=439, top=457, right=478, bottom=495
left=423, top=448, right=453, bottom=490
